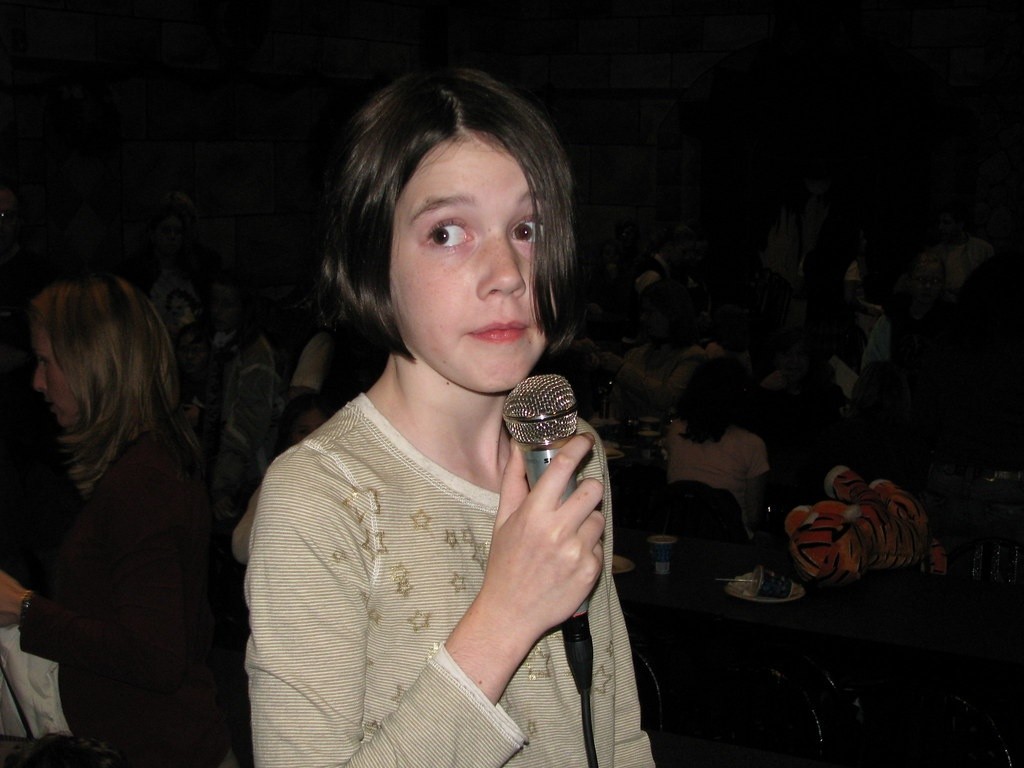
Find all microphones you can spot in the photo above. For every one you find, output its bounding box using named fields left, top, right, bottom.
left=502, top=373, right=595, bottom=687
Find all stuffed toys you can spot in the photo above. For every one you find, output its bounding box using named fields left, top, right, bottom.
left=784, top=465, right=947, bottom=577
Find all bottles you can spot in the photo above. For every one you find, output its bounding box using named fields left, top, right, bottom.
left=597, top=393, right=610, bottom=419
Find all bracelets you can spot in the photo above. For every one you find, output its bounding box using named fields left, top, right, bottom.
left=20, top=590, right=32, bottom=623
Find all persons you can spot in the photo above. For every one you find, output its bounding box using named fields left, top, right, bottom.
left=243, top=65, right=656, bottom=768
left=0, top=191, right=1023, bottom=768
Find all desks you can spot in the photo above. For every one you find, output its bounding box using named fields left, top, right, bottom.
left=613, top=476, right=1024, bottom=768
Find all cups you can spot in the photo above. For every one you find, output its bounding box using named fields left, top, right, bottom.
left=647, top=535, right=677, bottom=575
left=750, top=566, right=793, bottom=599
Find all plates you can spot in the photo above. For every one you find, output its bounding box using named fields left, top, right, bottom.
left=640, top=416, right=659, bottom=423
left=638, top=431, right=660, bottom=436
left=724, top=584, right=805, bottom=603
left=611, top=554, right=635, bottom=575
left=606, top=448, right=625, bottom=460
